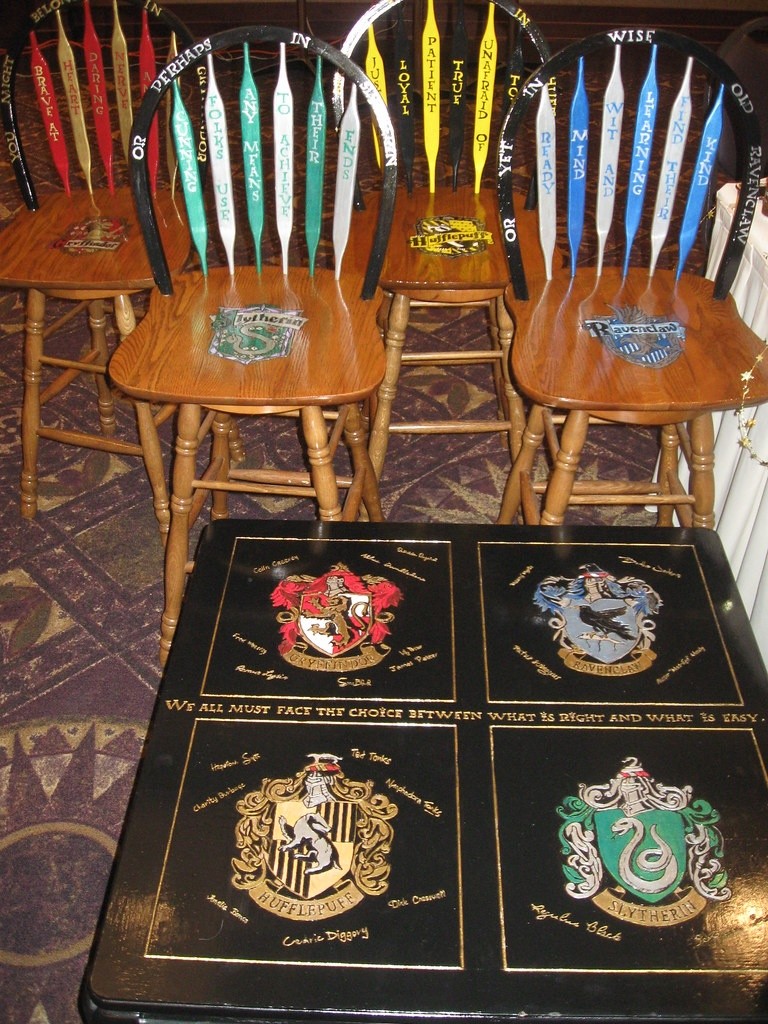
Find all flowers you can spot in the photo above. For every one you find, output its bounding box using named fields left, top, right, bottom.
left=699, top=205, right=717, bottom=224
left=734, top=346, right=767, bottom=467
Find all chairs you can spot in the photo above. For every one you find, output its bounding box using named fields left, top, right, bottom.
left=496, top=26, right=768, bottom=526
left=110, top=24, right=385, bottom=671
left=0, top=1, right=244, bottom=543
left=330, top=0, right=552, bottom=486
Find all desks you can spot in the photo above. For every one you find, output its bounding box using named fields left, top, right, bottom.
left=644, top=182, right=768, bottom=671
left=79, top=521, right=768, bottom=1024
